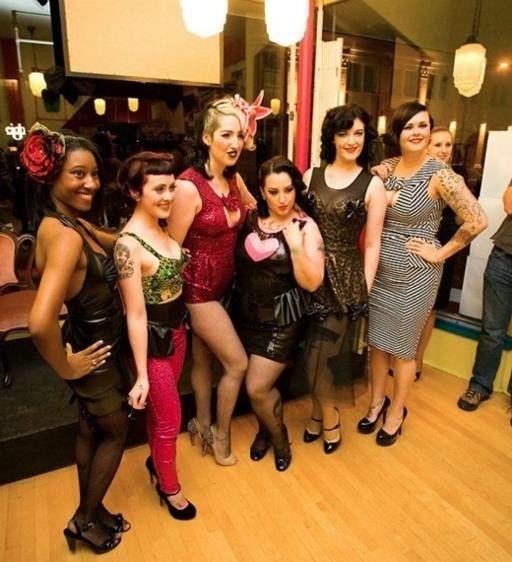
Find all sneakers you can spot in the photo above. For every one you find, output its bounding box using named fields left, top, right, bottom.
left=457, top=387, right=490, bottom=411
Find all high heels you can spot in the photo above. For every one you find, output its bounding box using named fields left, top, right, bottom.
left=357, top=395, right=391, bottom=434
left=322, top=407, right=341, bottom=455
left=156, top=482, right=196, bottom=520
left=201, top=423, right=239, bottom=466
left=388, top=368, right=394, bottom=377
left=250, top=431, right=272, bottom=461
left=413, top=371, right=422, bottom=382
left=187, top=417, right=213, bottom=456
left=64, top=514, right=121, bottom=554
left=303, top=416, right=323, bottom=443
left=146, top=455, right=159, bottom=484
left=376, top=406, right=408, bottom=447
left=272, top=424, right=292, bottom=472
left=95, top=505, right=131, bottom=532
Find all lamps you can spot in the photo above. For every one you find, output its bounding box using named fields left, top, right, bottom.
left=451, top=1, right=488, bottom=98
left=181, top=0, right=228, bottom=37
left=25, top=25, right=48, bottom=98
left=262, top=0, right=308, bottom=48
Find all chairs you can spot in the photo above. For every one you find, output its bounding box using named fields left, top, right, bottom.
left=0, top=223, right=70, bottom=386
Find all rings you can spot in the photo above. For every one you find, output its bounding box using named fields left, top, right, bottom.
left=91, top=360, right=97, bottom=365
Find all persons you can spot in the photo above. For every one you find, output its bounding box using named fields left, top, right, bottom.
left=231, top=155, right=325, bottom=472
left=457, top=178, right=512, bottom=427
left=166, top=89, right=308, bottom=467
left=356, top=100, right=489, bottom=447
left=301, top=104, right=387, bottom=453
left=389, top=126, right=453, bottom=382
left=111, top=151, right=197, bottom=520
left=16, top=122, right=132, bottom=555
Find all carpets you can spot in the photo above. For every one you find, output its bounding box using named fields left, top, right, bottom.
left=2, top=344, right=225, bottom=441
